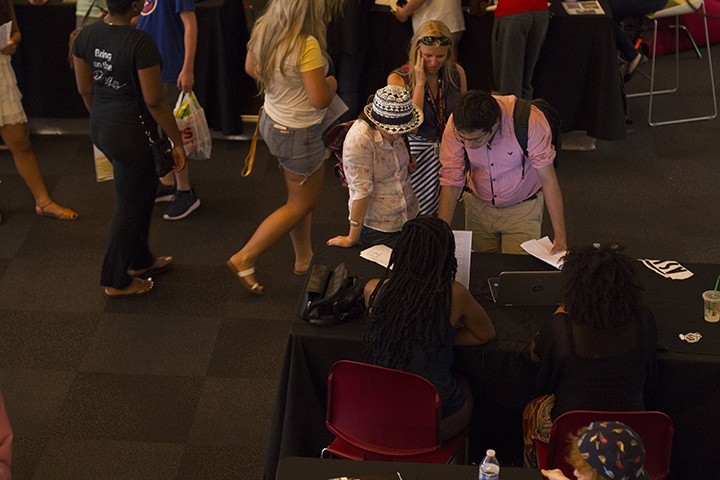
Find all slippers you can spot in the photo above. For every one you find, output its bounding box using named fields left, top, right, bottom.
left=105, top=277, right=155, bottom=296
left=129, top=256, right=172, bottom=280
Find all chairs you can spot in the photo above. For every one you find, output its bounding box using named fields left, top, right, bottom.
left=618, top=0, right=719, bottom=126
left=321, top=360, right=470, bottom=466
left=534, top=408, right=672, bottom=480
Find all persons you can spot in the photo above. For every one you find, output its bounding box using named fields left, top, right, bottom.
left=1, top=1, right=80, bottom=224
left=610, top=0, right=671, bottom=122
left=225, top=0, right=339, bottom=297
left=358, top=211, right=498, bottom=467
left=385, top=20, right=468, bottom=220
left=67, top=0, right=189, bottom=297
left=390, top=0, right=467, bottom=55
left=491, top=0, right=554, bottom=102
left=135, top=1, right=202, bottom=220
left=76, top=0, right=110, bottom=39
left=327, top=85, right=426, bottom=256
left=520, top=241, right=660, bottom=470
left=437, top=89, right=571, bottom=259
left=541, top=421, right=651, bottom=480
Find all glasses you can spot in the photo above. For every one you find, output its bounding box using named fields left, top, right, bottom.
left=457, top=121, right=497, bottom=143
left=416, top=36, right=453, bottom=46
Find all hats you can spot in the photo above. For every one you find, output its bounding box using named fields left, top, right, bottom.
left=578, top=420, right=650, bottom=480
left=363, top=85, right=424, bottom=135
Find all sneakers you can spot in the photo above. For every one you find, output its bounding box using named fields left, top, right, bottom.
left=164, top=185, right=203, bottom=221
left=153, top=183, right=178, bottom=203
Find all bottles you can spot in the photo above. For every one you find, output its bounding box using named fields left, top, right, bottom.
left=478, top=449, right=500, bottom=480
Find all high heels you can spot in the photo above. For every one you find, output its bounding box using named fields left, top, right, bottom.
left=623, top=54, right=648, bottom=84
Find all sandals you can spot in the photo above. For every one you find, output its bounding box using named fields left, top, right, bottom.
left=35, top=199, right=79, bottom=220
left=227, top=259, right=265, bottom=296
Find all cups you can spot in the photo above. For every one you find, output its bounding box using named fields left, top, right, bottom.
left=702, top=290, right=720, bottom=322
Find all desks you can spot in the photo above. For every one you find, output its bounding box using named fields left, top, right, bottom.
left=0, top=0, right=89, bottom=119
left=274, top=455, right=546, bottom=480
left=264, top=243, right=719, bottom=480
left=190, top=0, right=626, bottom=143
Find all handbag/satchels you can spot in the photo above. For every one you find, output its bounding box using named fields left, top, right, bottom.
left=171, top=88, right=213, bottom=160
left=151, top=135, right=176, bottom=177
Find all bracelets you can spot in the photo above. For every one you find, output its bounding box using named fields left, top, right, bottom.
left=349, top=219, right=367, bottom=228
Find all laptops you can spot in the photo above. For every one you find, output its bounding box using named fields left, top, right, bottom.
left=487, top=271, right=563, bottom=307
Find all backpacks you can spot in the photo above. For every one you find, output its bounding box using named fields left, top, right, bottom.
left=460, top=91, right=564, bottom=169
left=324, top=117, right=412, bottom=191
